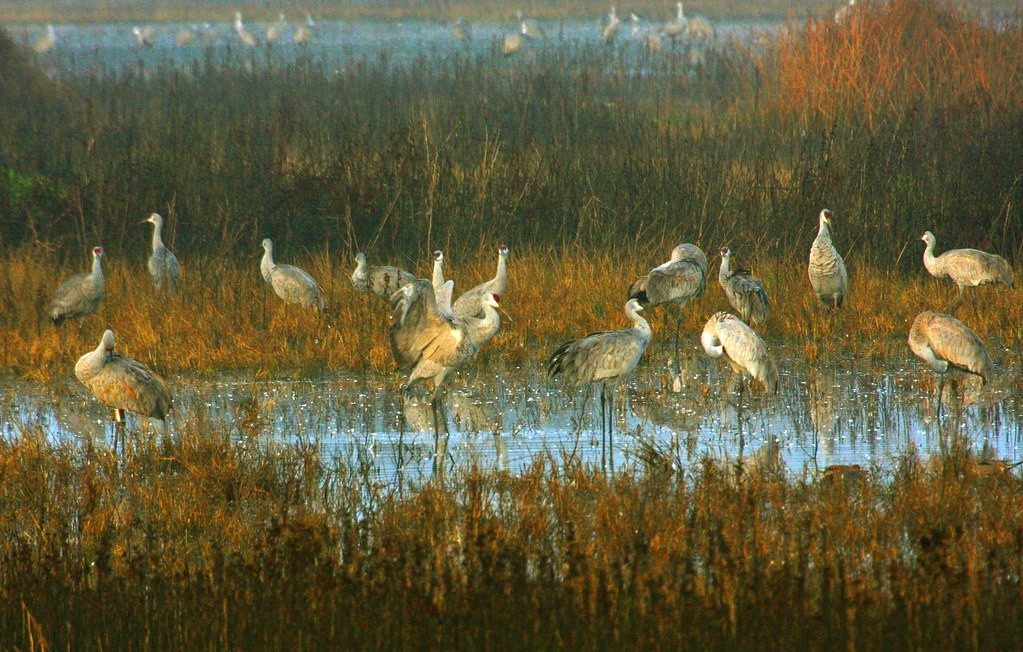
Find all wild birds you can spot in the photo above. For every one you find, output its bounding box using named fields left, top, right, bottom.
left=132, top=212, right=179, bottom=298
left=546, top=298, right=662, bottom=400
left=47, top=247, right=105, bottom=339
left=907, top=310, right=996, bottom=406
left=34, top=0, right=712, bottom=72
left=351, top=251, right=416, bottom=301
left=808, top=207, right=847, bottom=311
left=431, top=251, right=455, bottom=312
left=74, top=330, right=173, bottom=430
left=389, top=279, right=513, bottom=400
left=627, top=241, right=708, bottom=350
left=914, top=230, right=1018, bottom=315
left=710, top=246, right=772, bottom=327
left=450, top=244, right=510, bottom=318
left=258, top=237, right=325, bottom=315
left=699, top=312, right=781, bottom=398
